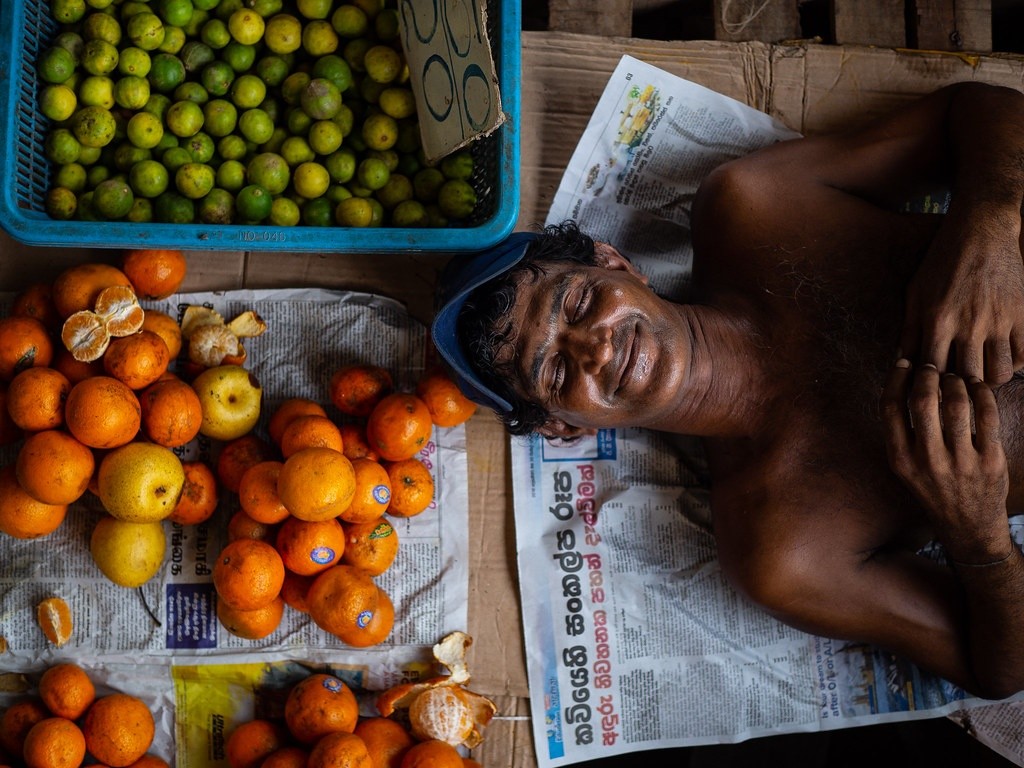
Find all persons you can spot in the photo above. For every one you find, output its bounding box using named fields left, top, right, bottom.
left=427, top=80, right=1023, bottom=700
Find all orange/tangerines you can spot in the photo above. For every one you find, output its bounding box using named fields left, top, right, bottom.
left=0, top=0, right=492, bottom=768
